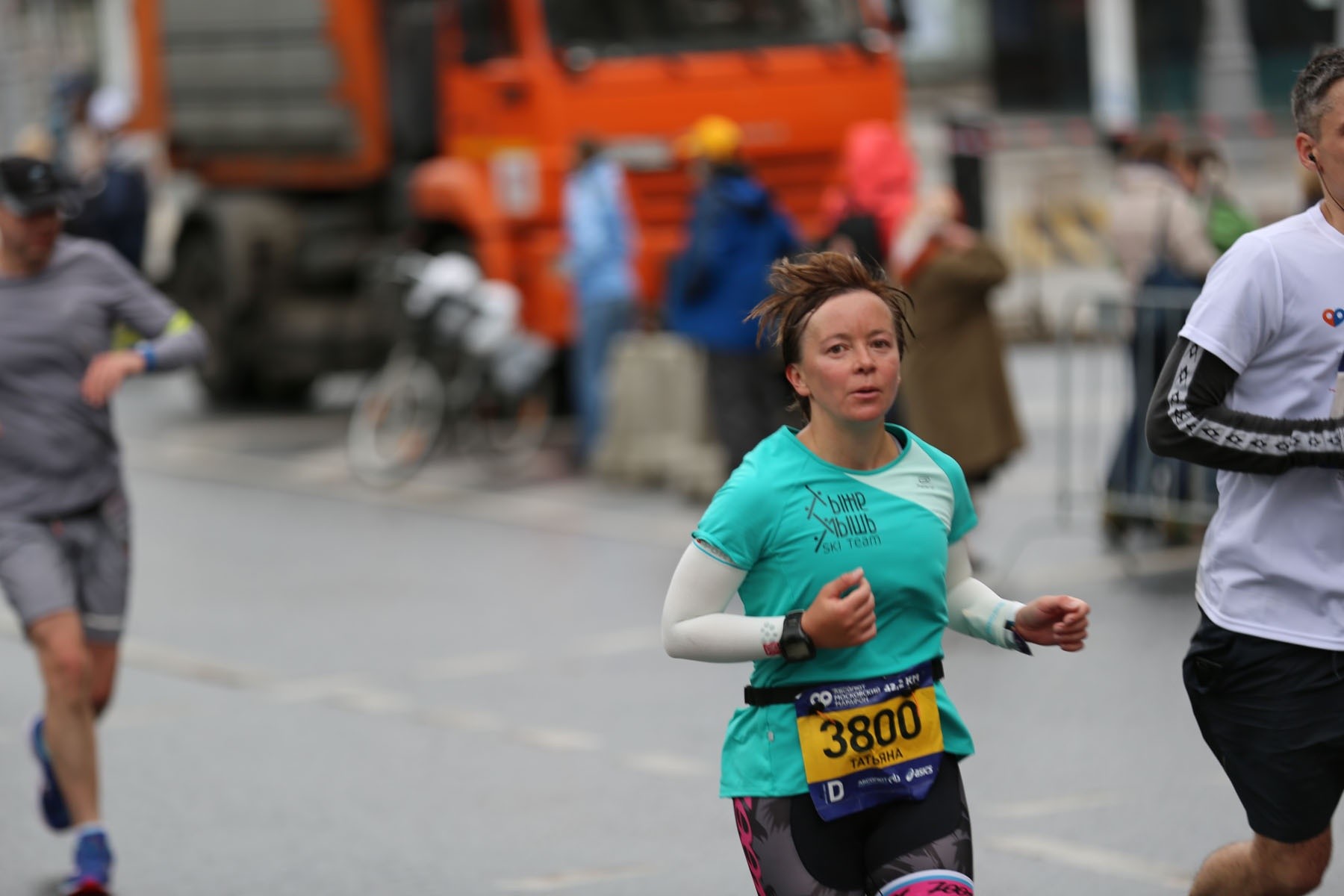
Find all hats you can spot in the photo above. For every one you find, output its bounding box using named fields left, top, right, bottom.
left=678, top=118, right=745, bottom=160
left=0, top=158, right=83, bottom=203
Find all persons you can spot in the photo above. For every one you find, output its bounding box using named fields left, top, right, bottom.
left=1144, top=46, right=1344, bottom=896
left=558, top=117, right=1241, bottom=462
left=1, top=155, right=215, bottom=896
left=661, top=249, right=1090, bottom=896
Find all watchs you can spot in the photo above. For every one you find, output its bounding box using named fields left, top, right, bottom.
left=778, top=609, right=817, bottom=664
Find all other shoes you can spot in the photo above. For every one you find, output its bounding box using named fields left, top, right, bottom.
left=63, top=832, right=113, bottom=896
left=32, top=719, right=73, bottom=829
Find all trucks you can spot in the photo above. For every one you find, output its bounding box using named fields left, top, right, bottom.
left=74, top=0, right=990, bottom=412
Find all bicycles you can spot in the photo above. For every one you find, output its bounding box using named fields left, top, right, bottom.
left=335, top=247, right=587, bottom=498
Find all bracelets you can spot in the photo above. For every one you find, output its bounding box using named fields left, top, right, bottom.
left=131, top=344, right=160, bottom=373
